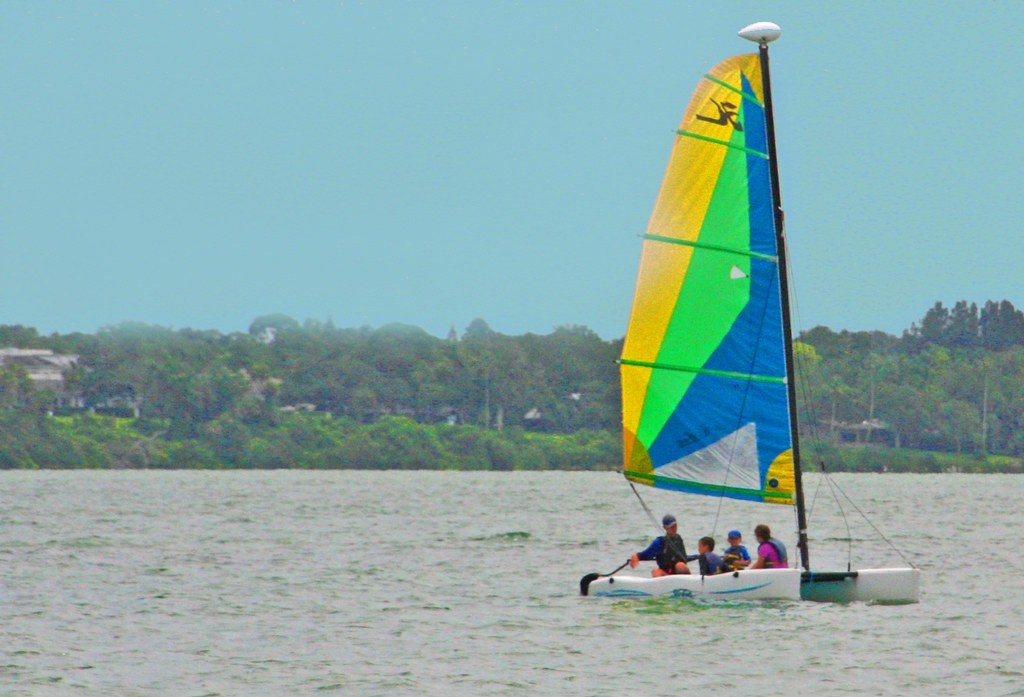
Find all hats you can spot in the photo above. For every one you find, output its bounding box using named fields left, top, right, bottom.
left=728, top=530, right=741, bottom=538
left=662, top=514, right=677, bottom=528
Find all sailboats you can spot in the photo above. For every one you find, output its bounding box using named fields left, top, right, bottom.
left=577, top=23, right=919, bottom=603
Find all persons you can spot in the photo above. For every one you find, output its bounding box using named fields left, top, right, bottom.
left=631, top=514, right=691, bottom=577
left=723, top=530, right=750, bottom=571
left=745, top=525, right=788, bottom=570
left=698, top=537, right=725, bottom=575
